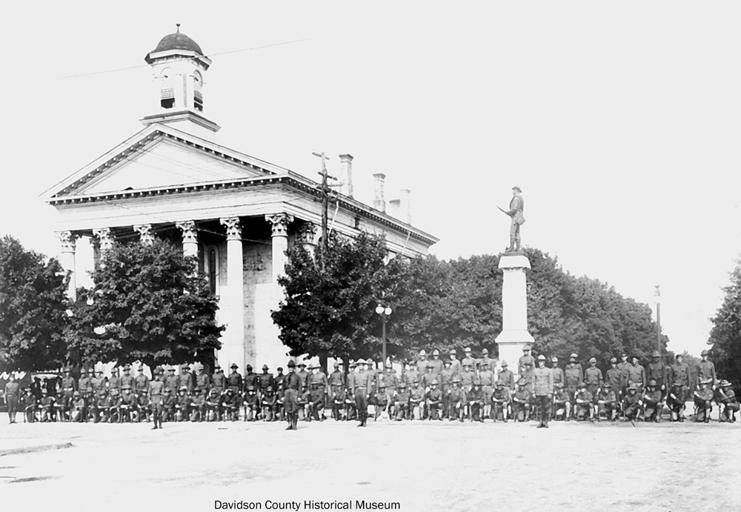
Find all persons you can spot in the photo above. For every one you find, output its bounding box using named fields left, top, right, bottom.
left=507, top=185, right=527, bottom=253
left=0, top=344, right=741, bottom=430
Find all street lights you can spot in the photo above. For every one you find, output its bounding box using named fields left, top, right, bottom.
left=374, top=304, right=392, bottom=370
left=654, top=284, right=663, bottom=354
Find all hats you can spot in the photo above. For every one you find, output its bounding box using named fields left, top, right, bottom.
left=64, top=366, right=143, bottom=373
left=24, top=384, right=132, bottom=398
left=609, top=351, right=659, bottom=363
left=523, top=346, right=597, bottom=366
left=671, top=378, right=733, bottom=388
left=246, top=360, right=322, bottom=371
left=701, top=350, right=710, bottom=356
left=247, top=386, right=274, bottom=392
left=163, top=385, right=234, bottom=394
left=537, top=412, right=550, bottom=429
left=557, top=379, right=657, bottom=391
left=333, top=358, right=373, bottom=368
left=378, top=377, right=527, bottom=389
left=419, top=348, right=508, bottom=369
left=153, top=362, right=239, bottom=375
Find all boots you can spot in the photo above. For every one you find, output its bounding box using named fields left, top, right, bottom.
left=285, top=414, right=297, bottom=430
left=152, top=415, right=162, bottom=429
left=358, top=413, right=367, bottom=428
